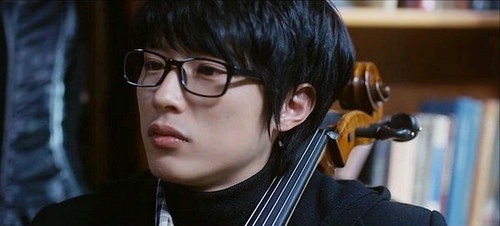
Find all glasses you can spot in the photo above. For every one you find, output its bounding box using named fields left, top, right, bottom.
left=122, top=47, right=266, bottom=97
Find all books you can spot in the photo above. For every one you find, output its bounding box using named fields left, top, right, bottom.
left=340, top=93, right=500, bottom=226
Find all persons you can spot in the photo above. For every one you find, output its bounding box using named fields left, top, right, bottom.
left=28, top=0, right=450, bottom=226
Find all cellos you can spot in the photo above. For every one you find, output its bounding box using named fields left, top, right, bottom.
left=233, top=59, right=421, bottom=226
